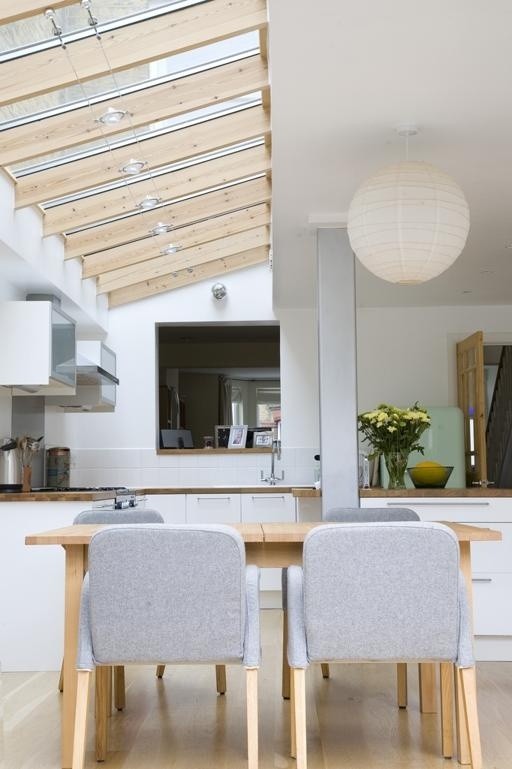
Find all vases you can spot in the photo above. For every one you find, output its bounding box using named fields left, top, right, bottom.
left=383, top=452, right=408, bottom=490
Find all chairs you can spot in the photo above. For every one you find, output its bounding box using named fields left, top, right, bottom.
left=60, top=507, right=227, bottom=712
left=72, top=523, right=263, bottom=766
left=287, top=522, right=484, bottom=767
left=281, top=507, right=420, bottom=708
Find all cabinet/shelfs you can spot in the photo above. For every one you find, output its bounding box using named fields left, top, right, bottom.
left=144, top=493, right=297, bottom=526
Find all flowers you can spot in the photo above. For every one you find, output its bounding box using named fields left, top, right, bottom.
left=358, top=401, right=432, bottom=484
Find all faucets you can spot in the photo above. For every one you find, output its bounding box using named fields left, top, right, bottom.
left=271, top=440, right=281, bottom=474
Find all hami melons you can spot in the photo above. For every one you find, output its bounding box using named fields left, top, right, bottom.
left=413, top=461, right=448, bottom=484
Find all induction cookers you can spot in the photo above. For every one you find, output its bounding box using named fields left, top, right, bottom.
left=32, top=485, right=126, bottom=495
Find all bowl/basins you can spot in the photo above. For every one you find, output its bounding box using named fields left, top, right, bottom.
left=405, top=465, right=454, bottom=491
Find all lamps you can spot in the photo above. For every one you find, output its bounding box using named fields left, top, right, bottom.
left=343, top=126, right=470, bottom=286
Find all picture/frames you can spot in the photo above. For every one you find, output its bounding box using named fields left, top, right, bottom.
left=252, top=431, right=272, bottom=448
left=227, top=424, right=248, bottom=449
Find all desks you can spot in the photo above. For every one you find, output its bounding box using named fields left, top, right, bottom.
left=25, top=519, right=503, bottom=767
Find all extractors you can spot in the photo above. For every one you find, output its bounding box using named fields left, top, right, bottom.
left=74, top=351, right=120, bottom=388
